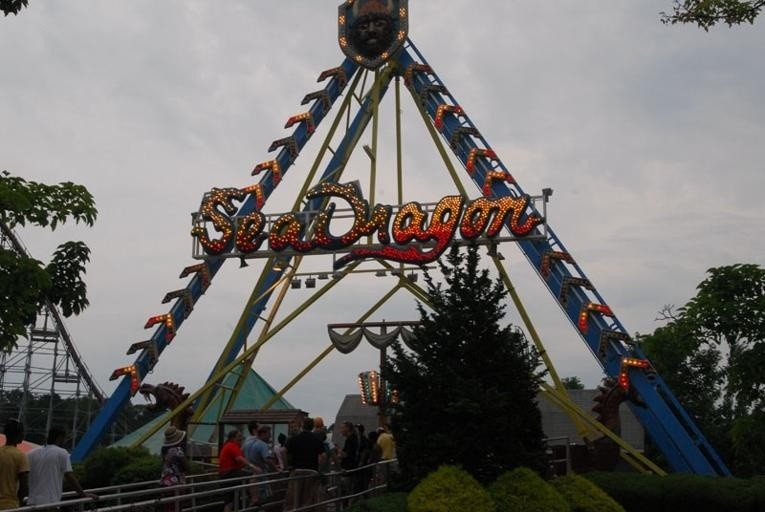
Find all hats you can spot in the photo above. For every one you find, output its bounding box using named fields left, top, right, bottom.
left=162, top=426, right=186, bottom=447
left=314, top=417, right=323, bottom=426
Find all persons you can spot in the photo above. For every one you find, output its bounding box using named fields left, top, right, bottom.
left=217, top=419, right=396, bottom=511
left=24, top=423, right=99, bottom=511
left=0, top=418, right=29, bottom=509
left=550, top=447, right=566, bottom=457
left=158, top=427, right=191, bottom=512
left=352, top=0, right=394, bottom=58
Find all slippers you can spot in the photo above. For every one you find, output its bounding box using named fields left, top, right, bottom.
left=248, top=498, right=272, bottom=507
